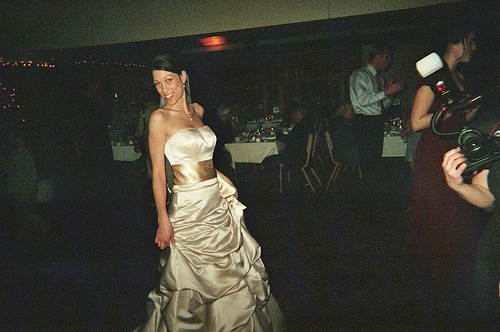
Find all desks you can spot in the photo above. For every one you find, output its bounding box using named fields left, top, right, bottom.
left=382, top=132, right=407, bottom=157
left=224, top=142, right=279, bottom=195
left=112, top=146, right=141, bottom=161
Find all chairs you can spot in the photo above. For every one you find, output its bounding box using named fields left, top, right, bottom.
left=325, top=131, right=361, bottom=191
left=279, top=130, right=324, bottom=194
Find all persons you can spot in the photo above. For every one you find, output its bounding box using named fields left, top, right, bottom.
left=408, top=23, right=487, bottom=286
left=207, top=102, right=353, bottom=184
left=441, top=147, right=500, bottom=332
left=77, top=79, right=113, bottom=120
left=0, top=116, right=49, bottom=241
left=349, top=45, right=403, bottom=186
left=134, top=54, right=287, bottom=332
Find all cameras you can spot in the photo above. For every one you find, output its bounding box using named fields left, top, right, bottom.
left=416, top=51, right=500, bottom=176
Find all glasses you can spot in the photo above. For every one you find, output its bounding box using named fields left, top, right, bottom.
left=383, top=55, right=392, bottom=60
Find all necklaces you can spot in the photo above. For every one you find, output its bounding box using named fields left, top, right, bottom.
left=185, top=112, right=192, bottom=120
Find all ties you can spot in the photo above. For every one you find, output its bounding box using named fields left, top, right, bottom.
left=376, top=72, right=384, bottom=114
